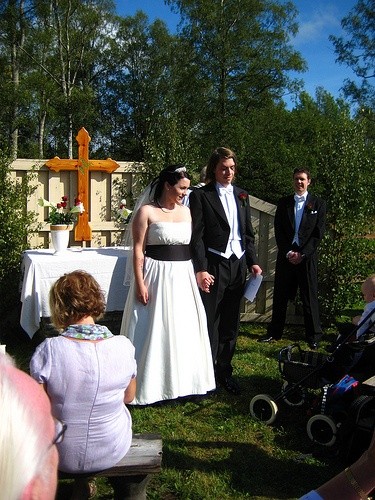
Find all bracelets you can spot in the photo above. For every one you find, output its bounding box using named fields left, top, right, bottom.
left=344, top=466, right=372, bottom=500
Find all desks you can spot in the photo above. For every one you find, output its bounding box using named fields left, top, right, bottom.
left=21, top=246, right=133, bottom=338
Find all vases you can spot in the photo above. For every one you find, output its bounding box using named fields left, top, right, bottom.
left=50, top=224, right=71, bottom=255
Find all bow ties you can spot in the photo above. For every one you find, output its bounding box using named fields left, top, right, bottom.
left=294, top=195, right=305, bottom=201
left=219, top=186, right=233, bottom=195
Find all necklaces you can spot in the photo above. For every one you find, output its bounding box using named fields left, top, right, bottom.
left=156, top=200, right=177, bottom=213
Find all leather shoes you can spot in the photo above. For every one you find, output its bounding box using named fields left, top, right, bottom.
left=217, top=376, right=240, bottom=394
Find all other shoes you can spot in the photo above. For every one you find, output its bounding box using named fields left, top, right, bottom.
left=258, top=336, right=274, bottom=342
left=310, top=341, right=318, bottom=348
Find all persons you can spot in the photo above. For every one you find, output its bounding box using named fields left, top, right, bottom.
left=30, top=269, right=138, bottom=500
left=189, top=147, right=262, bottom=396
left=353, top=273, right=375, bottom=339
left=257, top=165, right=327, bottom=351
left=0, top=351, right=67, bottom=500
left=120, top=163, right=215, bottom=406
left=298, top=430, right=375, bottom=500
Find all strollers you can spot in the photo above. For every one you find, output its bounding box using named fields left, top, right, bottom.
left=249, top=307, right=375, bottom=448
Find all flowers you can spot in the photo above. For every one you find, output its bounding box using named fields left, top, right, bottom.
left=306, top=201, right=317, bottom=215
left=238, top=192, right=249, bottom=208
left=112, top=199, right=133, bottom=223
left=36, top=195, right=85, bottom=227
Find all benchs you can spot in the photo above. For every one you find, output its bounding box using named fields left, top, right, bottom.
left=58, top=433, right=163, bottom=500
